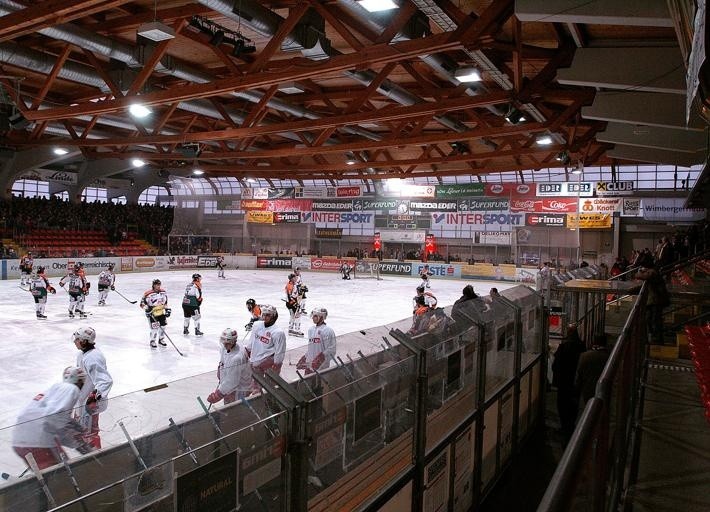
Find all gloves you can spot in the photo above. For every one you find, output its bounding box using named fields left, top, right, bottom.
left=223, top=264, right=227, bottom=267
left=198, top=299, right=203, bottom=307
left=266, top=363, right=282, bottom=385
left=292, top=302, right=299, bottom=311
left=83, top=290, right=90, bottom=296
left=86, top=389, right=102, bottom=419
left=110, top=285, right=116, bottom=291
left=245, top=322, right=254, bottom=333
left=59, top=281, right=66, bottom=287
left=144, top=309, right=155, bottom=319
left=312, top=353, right=323, bottom=371
left=49, top=287, right=56, bottom=296
left=300, top=286, right=309, bottom=295
left=295, top=356, right=300, bottom=370
left=207, top=390, right=224, bottom=404
left=164, top=307, right=172, bottom=317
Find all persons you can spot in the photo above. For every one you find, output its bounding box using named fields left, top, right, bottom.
left=339, top=261, right=352, bottom=280
left=405, top=296, right=433, bottom=338
left=421, top=264, right=430, bottom=288
left=10, top=365, right=88, bottom=473
left=29, top=265, right=56, bottom=320
left=578, top=338, right=610, bottom=402
left=20, top=251, right=33, bottom=284
left=97, top=265, right=115, bottom=304
left=470, top=255, right=475, bottom=262
left=140, top=278, right=171, bottom=349
left=429, top=307, right=454, bottom=335
left=294, top=267, right=308, bottom=317
left=205, top=328, right=251, bottom=406
left=60, top=266, right=91, bottom=319
left=13, top=194, right=209, bottom=257
left=244, top=298, right=262, bottom=331
left=296, top=307, right=336, bottom=377
left=71, top=326, right=113, bottom=452
left=182, top=272, right=205, bottom=335
left=541, top=263, right=549, bottom=271
left=217, top=256, right=226, bottom=278
left=636, top=260, right=664, bottom=344
left=75, top=264, right=89, bottom=313
left=451, top=285, right=487, bottom=321
left=284, top=274, right=305, bottom=338
left=412, top=285, right=437, bottom=321
left=242, top=305, right=286, bottom=395
left=631, top=247, right=650, bottom=266
left=347, top=247, right=460, bottom=262
left=551, top=322, right=585, bottom=434
left=486, top=289, right=500, bottom=307
left=659, top=237, right=672, bottom=263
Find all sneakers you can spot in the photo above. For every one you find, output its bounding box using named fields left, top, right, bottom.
left=101, top=300, right=106, bottom=304
left=219, top=275, right=221, bottom=277
left=296, top=332, right=304, bottom=336
left=221, top=275, right=225, bottom=278
left=302, top=309, right=307, bottom=314
left=195, top=329, right=203, bottom=335
left=36, top=313, right=47, bottom=318
left=21, top=281, right=26, bottom=286
left=98, top=300, right=102, bottom=304
left=158, top=338, right=168, bottom=346
left=150, top=340, right=157, bottom=347
left=69, top=311, right=75, bottom=317
left=183, top=329, right=189, bottom=334
left=346, top=276, right=350, bottom=280
left=342, top=275, right=346, bottom=279
left=75, top=309, right=81, bottom=313
left=288, top=329, right=297, bottom=335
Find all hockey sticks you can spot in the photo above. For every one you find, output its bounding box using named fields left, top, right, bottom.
left=150, top=314, right=188, bottom=358
left=0, top=464, right=32, bottom=483
left=62, top=285, right=90, bottom=314
left=113, top=288, right=138, bottom=305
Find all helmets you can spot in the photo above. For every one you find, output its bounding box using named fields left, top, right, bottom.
left=38, top=265, right=45, bottom=270
left=28, top=251, right=32, bottom=255
left=288, top=273, right=295, bottom=281
left=424, top=263, right=429, bottom=267
left=220, top=328, right=239, bottom=344
left=71, top=326, right=96, bottom=345
left=413, top=295, right=424, bottom=303
left=309, top=307, right=328, bottom=321
left=63, top=366, right=87, bottom=384
left=73, top=262, right=84, bottom=273
left=109, top=264, right=115, bottom=268
left=261, top=305, right=279, bottom=327
left=246, top=299, right=255, bottom=311
left=152, top=278, right=160, bottom=284
left=416, top=287, right=425, bottom=293
left=192, top=273, right=201, bottom=279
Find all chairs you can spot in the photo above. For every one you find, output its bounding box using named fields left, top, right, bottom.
left=685, top=323, right=710, bottom=425
left=695, top=259, right=710, bottom=275
left=671, top=273, right=693, bottom=288
left=0, top=227, right=164, bottom=258
